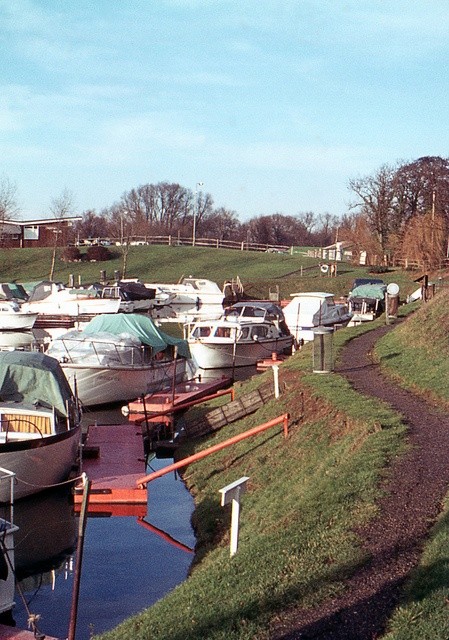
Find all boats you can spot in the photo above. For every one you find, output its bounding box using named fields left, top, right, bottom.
left=185, top=302, right=294, bottom=369
left=0, top=279, right=227, bottom=348
left=44, top=313, right=190, bottom=407
left=0, top=349, right=84, bottom=503
left=282, top=293, right=354, bottom=326
left=348, top=283, right=387, bottom=322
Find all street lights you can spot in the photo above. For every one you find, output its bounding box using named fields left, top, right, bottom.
left=192, top=183, right=204, bottom=247
